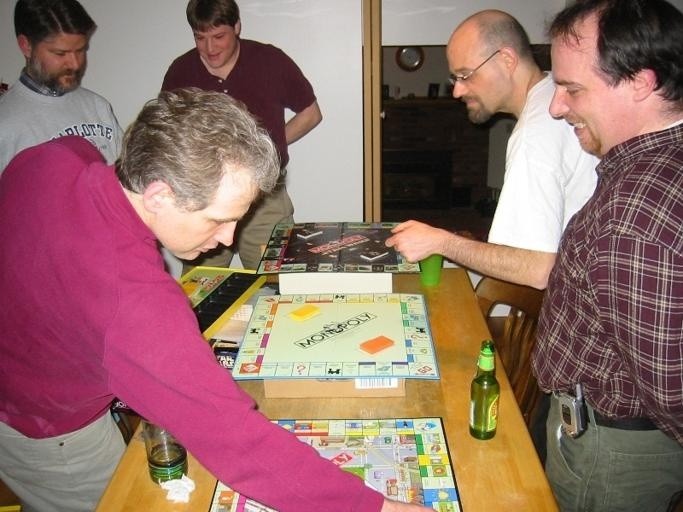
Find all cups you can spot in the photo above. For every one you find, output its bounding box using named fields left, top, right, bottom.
left=419, top=253, right=442, bottom=287
left=138, top=418, right=188, bottom=488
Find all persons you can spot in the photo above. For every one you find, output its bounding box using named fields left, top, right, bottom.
left=385, top=10, right=602, bottom=472
left=530, top=0, right=683, bottom=512
left=0, top=0, right=125, bottom=177
left=0, top=87, right=440, bottom=512
left=158, top=0, right=323, bottom=280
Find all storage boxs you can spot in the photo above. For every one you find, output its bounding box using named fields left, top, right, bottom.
left=277, top=269, right=394, bottom=296
left=263, top=372, right=407, bottom=398
left=211, top=338, right=239, bottom=368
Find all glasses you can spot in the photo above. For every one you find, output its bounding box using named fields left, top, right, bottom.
left=448, top=50, right=500, bottom=85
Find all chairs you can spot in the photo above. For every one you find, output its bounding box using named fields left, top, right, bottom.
left=473, top=271, right=552, bottom=435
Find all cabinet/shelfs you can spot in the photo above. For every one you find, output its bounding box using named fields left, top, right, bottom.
left=380, top=99, right=488, bottom=236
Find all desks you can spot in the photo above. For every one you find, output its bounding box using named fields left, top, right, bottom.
left=96, top=265, right=562, bottom=512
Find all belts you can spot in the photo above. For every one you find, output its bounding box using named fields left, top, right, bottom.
left=584, top=406, right=659, bottom=432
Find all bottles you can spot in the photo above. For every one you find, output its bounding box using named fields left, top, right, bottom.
left=468, top=339, right=500, bottom=440
